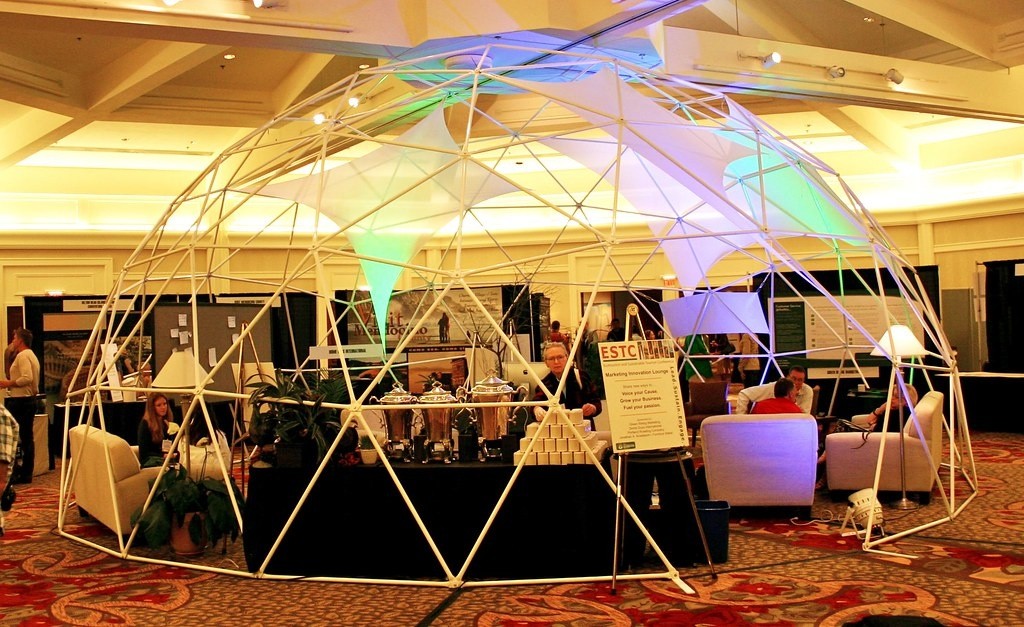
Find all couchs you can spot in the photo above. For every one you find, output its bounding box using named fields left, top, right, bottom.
left=700, top=413, right=818, bottom=520
left=69, top=424, right=221, bottom=544
left=825, top=391, right=944, bottom=503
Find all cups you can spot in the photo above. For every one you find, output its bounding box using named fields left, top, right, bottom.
left=513, top=409, right=608, bottom=466
left=858, top=384, right=865, bottom=392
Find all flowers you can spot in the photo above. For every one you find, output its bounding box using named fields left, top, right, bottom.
left=130, top=422, right=244, bottom=549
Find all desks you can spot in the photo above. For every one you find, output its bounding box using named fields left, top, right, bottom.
left=33, top=414, right=50, bottom=476
left=243, top=447, right=621, bottom=580
left=52, top=398, right=175, bottom=460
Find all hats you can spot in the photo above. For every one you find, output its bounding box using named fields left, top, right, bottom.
left=612, top=318, right=619, bottom=325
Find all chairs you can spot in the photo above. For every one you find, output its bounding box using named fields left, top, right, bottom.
left=685, top=381, right=730, bottom=446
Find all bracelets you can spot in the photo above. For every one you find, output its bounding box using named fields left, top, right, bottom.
left=873, top=411, right=879, bottom=416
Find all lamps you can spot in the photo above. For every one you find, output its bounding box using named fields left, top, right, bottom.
left=888, top=67, right=905, bottom=84
left=827, top=66, right=846, bottom=79
left=848, top=487, right=884, bottom=531
left=254, top=0, right=282, bottom=9
left=763, top=52, right=781, bottom=69
left=869, top=324, right=929, bottom=510
left=151, top=350, right=214, bottom=478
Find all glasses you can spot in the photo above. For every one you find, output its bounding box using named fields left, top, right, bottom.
left=545, top=354, right=566, bottom=362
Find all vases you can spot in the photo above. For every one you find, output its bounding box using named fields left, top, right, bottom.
left=169, top=512, right=208, bottom=559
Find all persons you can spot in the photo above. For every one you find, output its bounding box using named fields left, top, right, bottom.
left=815, top=383, right=918, bottom=490
left=0, top=328, right=40, bottom=484
left=438, top=313, right=450, bottom=344
left=138, top=392, right=180, bottom=467
left=189, top=401, right=228, bottom=449
left=531, top=342, right=602, bottom=431
left=751, top=378, right=802, bottom=414
left=118, top=345, right=135, bottom=375
left=574, top=317, right=662, bottom=370
left=738, top=333, right=760, bottom=414
left=358, top=362, right=402, bottom=396
left=0, top=404, right=19, bottom=499
left=550, top=321, right=570, bottom=354
left=674, top=334, right=731, bottom=405
left=137, top=362, right=151, bottom=396
left=249, top=409, right=302, bottom=460
left=737, top=366, right=813, bottom=414
left=541, top=310, right=550, bottom=328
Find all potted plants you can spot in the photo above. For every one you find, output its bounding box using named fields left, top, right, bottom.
left=360, top=435, right=377, bottom=464
left=243, top=369, right=343, bottom=468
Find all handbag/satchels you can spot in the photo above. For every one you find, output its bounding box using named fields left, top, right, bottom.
left=836, top=419, right=869, bottom=432
left=721, top=340, right=735, bottom=354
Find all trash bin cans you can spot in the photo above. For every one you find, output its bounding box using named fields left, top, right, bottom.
left=691, top=500, right=730, bottom=565
